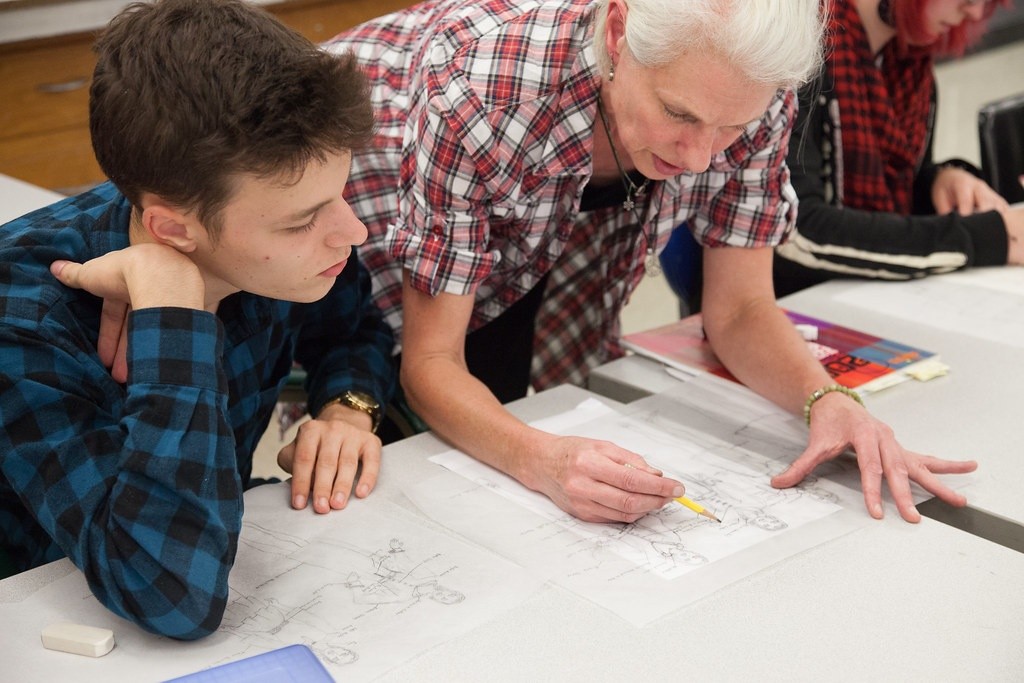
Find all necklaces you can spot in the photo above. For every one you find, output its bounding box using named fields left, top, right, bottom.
left=596, top=91, right=658, bottom=256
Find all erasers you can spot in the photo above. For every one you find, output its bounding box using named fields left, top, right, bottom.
left=40, top=623, right=114, bottom=658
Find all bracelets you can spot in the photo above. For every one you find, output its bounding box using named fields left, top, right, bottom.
left=803, top=385, right=865, bottom=427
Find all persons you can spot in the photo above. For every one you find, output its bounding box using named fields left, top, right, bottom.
left=277, top=0, right=979, bottom=522
left=0, top=0, right=400, bottom=640
left=660, top=0, right=1024, bottom=317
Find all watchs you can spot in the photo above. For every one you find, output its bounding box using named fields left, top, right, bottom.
left=318, top=389, right=383, bottom=432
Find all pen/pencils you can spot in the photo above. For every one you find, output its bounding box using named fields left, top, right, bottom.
left=624, top=463, right=722, bottom=523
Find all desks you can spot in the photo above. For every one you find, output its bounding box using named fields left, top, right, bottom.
left=0, top=261, right=1024, bottom=683
